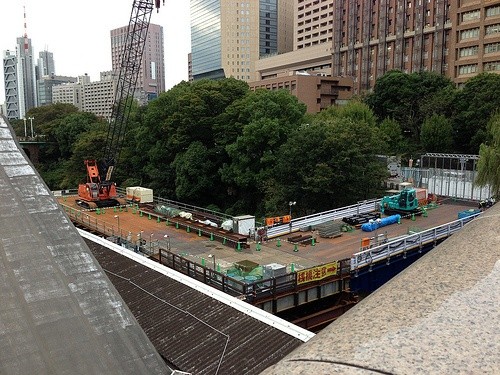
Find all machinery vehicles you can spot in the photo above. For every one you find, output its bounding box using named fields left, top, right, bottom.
left=75, top=0, right=165, bottom=213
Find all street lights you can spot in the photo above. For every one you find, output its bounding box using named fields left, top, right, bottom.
left=288, top=202, right=296, bottom=235
